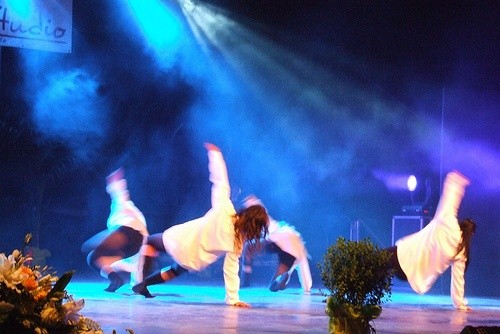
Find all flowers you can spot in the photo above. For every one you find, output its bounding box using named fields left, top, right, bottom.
left=0, top=233, right=135, bottom=334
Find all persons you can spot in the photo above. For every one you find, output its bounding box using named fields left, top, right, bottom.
left=81, top=168, right=150, bottom=292
left=132, top=143, right=271, bottom=307
left=322, top=171, right=477, bottom=310
left=239, top=194, right=313, bottom=293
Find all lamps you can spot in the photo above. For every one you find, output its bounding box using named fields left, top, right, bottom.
left=407, top=175, right=436, bottom=205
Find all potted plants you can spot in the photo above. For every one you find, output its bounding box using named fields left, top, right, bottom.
left=318, top=237, right=397, bottom=334
